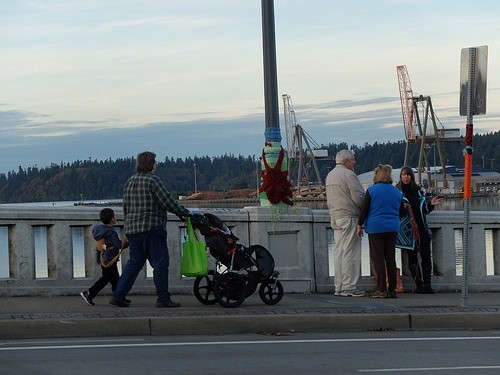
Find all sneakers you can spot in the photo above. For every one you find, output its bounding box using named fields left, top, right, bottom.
left=369, top=290, right=388, bottom=298
left=387, top=292, right=398, bottom=298
left=80, top=290, right=95, bottom=306
left=341, top=289, right=366, bottom=297
left=334, top=291, right=341, bottom=296
left=125, top=299, right=132, bottom=304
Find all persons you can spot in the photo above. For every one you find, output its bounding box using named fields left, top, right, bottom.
left=395, top=167, right=444, bottom=294
left=356, top=165, right=405, bottom=299
left=108, top=151, right=191, bottom=308
left=80, top=208, right=132, bottom=306
left=325, top=150, right=366, bottom=298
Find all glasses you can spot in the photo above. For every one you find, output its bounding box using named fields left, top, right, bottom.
left=379, top=164, right=382, bottom=169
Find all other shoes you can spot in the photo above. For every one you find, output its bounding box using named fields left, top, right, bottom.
left=417, top=287, right=425, bottom=293
left=425, top=288, right=435, bottom=294
left=108, top=297, right=129, bottom=307
left=156, top=299, right=181, bottom=308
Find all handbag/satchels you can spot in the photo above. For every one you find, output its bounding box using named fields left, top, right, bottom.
left=180, top=217, right=208, bottom=277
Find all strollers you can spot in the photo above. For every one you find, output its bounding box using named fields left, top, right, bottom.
left=180, top=213, right=284, bottom=308
